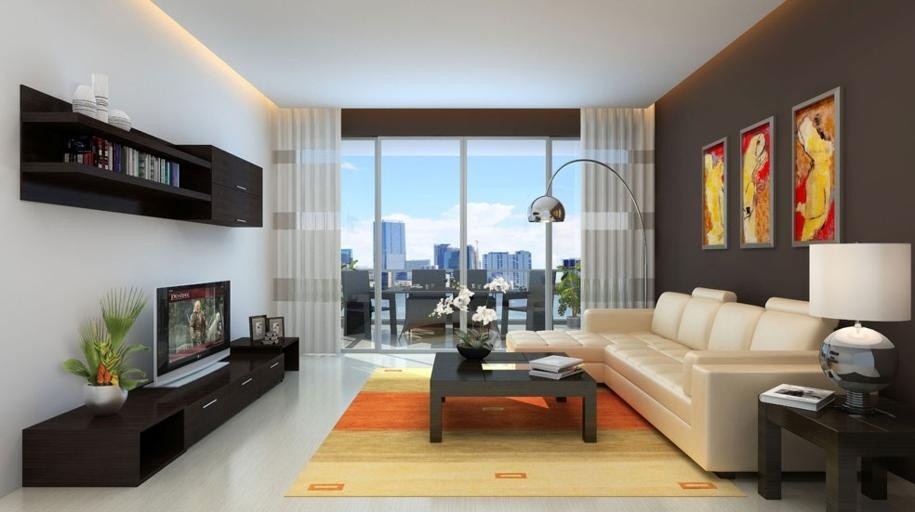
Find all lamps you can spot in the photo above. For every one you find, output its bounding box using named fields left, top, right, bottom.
left=804, top=236, right=915, bottom=415
left=525, top=159, right=648, bottom=309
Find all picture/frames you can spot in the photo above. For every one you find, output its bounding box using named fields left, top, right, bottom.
left=248, top=314, right=268, bottom=342
left=265, top=316, right=285, bottom=343
left=699, top=136, right=731, bottom=251
left=737, top=113, right=778, bottom=249
left=789, top=83, right=847, bottom=251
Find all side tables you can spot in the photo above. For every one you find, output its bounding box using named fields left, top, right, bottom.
left=750, top=384, right=912, bottom=509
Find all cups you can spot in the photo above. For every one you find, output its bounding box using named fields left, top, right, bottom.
left=398, top=263, right=439, bottom=290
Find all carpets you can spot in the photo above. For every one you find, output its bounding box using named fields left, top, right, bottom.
left=273, top=361, right=761, bottom=503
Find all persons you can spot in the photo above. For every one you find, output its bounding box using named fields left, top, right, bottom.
left=190, top=300, right=207, bottom=346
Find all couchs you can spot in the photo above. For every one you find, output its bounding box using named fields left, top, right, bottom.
left=575, top=282, right=855, bottom=485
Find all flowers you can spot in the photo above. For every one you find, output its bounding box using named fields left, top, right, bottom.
left=425, top=274, right=518, bottom=353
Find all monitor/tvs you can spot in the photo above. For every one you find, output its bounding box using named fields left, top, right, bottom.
left=144, top=280, right=230, bottom=389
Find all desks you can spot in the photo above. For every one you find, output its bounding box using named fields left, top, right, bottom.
left=232, top=335, right=302, bottom=372
left=418, top=345, right=606, bottom=446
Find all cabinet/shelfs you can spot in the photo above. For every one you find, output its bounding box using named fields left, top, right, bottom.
left=20, top=347, right=289, bottom=489
left=176, top=143, right=266, bottom=228
left=17, top=103, right=212, bottom=232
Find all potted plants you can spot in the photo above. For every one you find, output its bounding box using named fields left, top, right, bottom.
left=51, top=284, right=154, bottom=415
left=551, top=260, right=581, bottom=330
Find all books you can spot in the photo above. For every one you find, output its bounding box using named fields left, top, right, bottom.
left=530, top=367, right=586, bottom=380
left=529, top=355, right=585, bottom=373
left=63, top=137, right=179, bottom=188
left=759, top=383, right=836, bottom=411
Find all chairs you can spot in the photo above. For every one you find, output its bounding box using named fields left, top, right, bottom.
left=339, top=266, right=557, bottom=339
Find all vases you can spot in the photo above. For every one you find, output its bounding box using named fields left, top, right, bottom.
left=454, top=340, right=498, bottom=360
left=70, top=73, right=133, bottom=133
